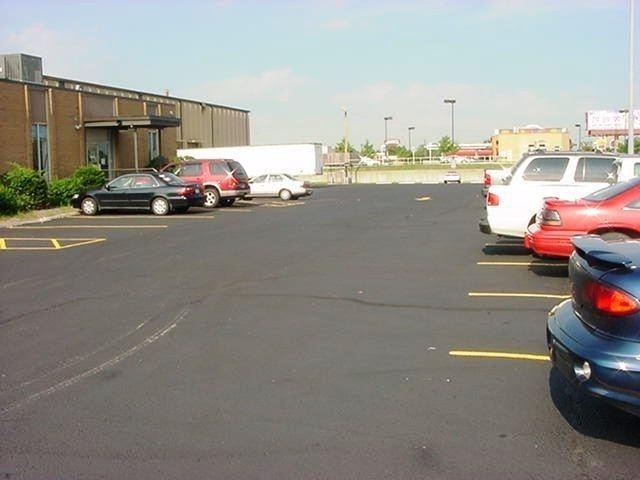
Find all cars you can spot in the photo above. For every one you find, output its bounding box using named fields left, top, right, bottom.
left=444, top=171, right=462, bottom=184
left=360, top=158, right=382, bottom=168
left=526, top=178, right=640, bottom=261
left=441, top=155, right=472, bottom=164
left=243, top=171, right=313, bottom=203
left=548, top=232, right=640, bottom=422
left=70, top=171, right=207, bottom=217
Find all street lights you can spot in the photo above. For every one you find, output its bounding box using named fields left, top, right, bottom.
left=444, top=98, right=457, bottom=145
left=408, top=126, right=415, bottom=150
left=619, top=109, right=629, bottom=140
left=341, top=107, right=350, bottom=153
left=384, top=116, right=393, bottom=141
left=575, top=123, right=582, bottom=144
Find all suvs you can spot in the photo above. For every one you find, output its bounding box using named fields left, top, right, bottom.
left=159, top=159, right=251, bottom=209
left=479, top=150, right=640, bottom=242
